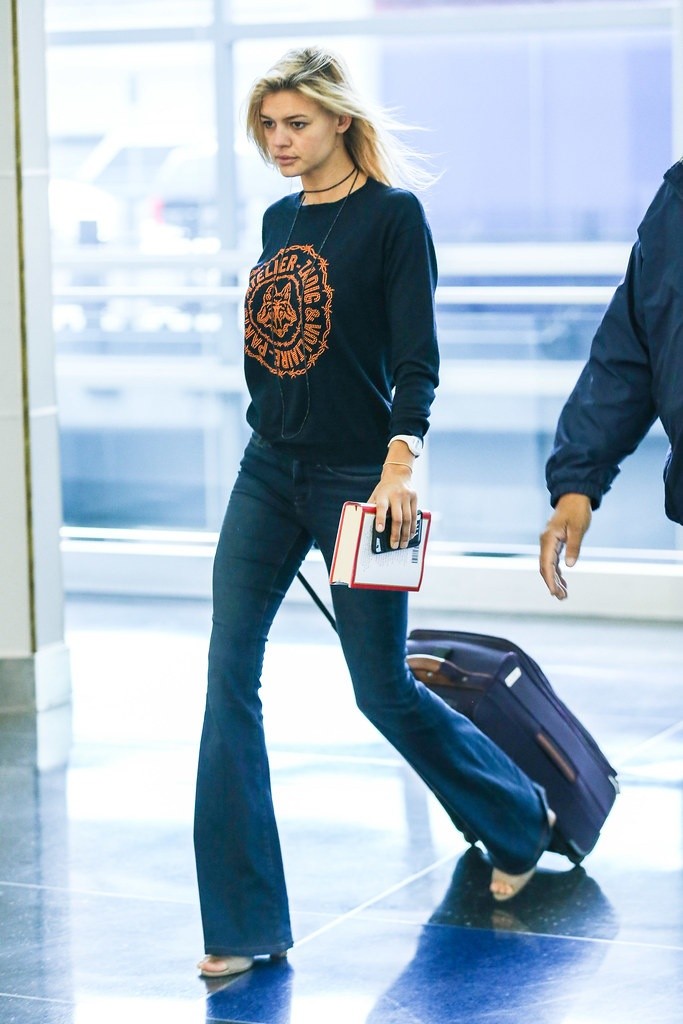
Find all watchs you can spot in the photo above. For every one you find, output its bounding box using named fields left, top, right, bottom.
left=385, top=430, right=422, bottom=460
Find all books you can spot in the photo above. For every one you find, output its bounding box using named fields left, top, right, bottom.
left=328, top=500, right=432, bottom=594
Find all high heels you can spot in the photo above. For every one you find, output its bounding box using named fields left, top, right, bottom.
left=200, top=949, right=287, bottom=978
left=490, top=808, right=557, bottom=902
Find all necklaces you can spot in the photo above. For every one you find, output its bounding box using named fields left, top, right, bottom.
left=272, top=165, right=361, bottom=438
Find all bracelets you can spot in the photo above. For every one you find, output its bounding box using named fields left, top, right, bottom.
left=382, top=462, right=414, bottom=474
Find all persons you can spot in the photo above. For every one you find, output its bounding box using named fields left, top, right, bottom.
left=536, top=145, right=683, bottom=603
left=192, top=46, right=558, bottom=982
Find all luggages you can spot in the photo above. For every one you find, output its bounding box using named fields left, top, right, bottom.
left=404, top=629, right=622, bottom=868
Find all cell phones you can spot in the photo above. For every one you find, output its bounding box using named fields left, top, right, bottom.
left=371, top=509, right=422, bottom=554
